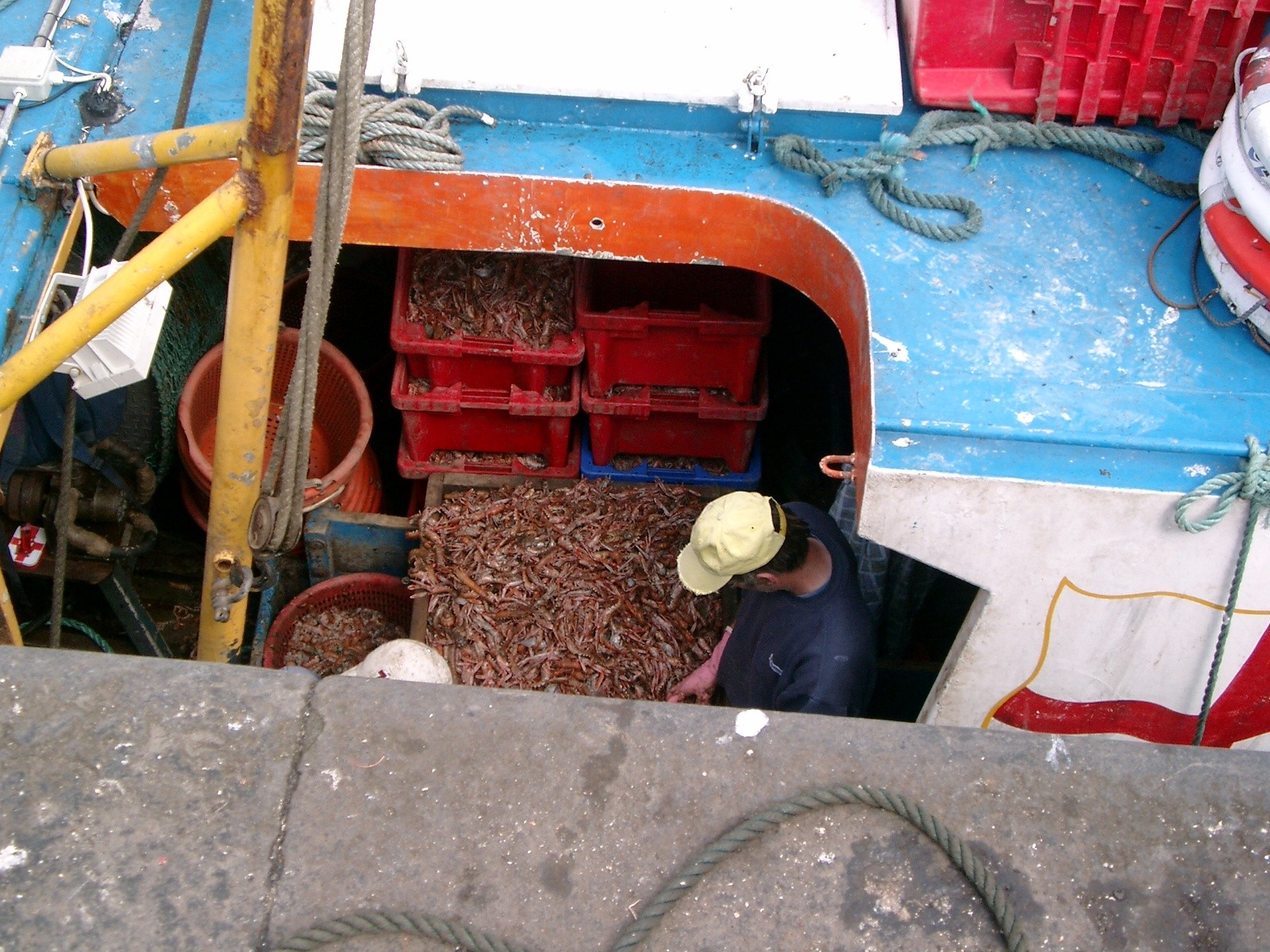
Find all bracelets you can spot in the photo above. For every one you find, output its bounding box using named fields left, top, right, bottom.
left=725, top=625, right=734, bottom=634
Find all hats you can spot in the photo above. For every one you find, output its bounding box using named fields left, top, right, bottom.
left=678, top=491, right=787, bottom=594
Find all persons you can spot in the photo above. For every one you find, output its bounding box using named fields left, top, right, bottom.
left=667, top=490, right=878, bottom=720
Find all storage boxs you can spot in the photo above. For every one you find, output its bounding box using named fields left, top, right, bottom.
left=390, top=244, right=771, bottom=489
